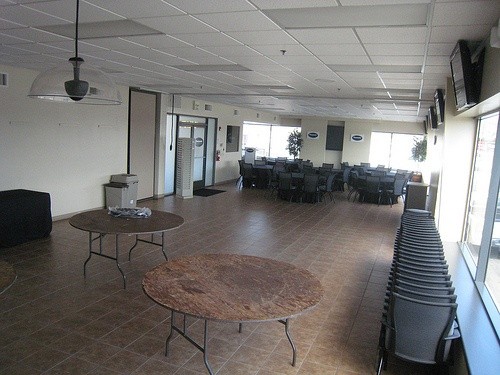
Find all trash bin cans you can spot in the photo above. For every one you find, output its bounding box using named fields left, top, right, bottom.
left=104, top=174, right=138, bottom=208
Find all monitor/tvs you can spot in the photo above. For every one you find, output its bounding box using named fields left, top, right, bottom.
left=449, top=40, right=486, bottom=112
left=423, top=89, right=446, bottom=134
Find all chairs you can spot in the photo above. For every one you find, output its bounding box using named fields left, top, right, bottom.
left=236, top=157, right=414, bottom=208
left=378, top=210, right=461, bottom=375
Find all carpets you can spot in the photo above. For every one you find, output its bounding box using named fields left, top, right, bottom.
left=194, top=188, right=226, bottom=197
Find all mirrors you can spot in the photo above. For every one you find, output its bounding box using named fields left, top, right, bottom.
left=226, top=125, right=241, bottom=152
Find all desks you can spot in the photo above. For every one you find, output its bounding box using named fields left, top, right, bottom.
left=406, top=179, right=429, bottom=209
left=69, top=209, right=184, bottom=289
left=359, top=174, right=392, bottom=182
left=315, top=169, right=343, bottom=172
left=142, top=251, right=324, bottom=375
left=291, top=173, right=305, bottom=178
left=254, top=164, right=273, bottom=168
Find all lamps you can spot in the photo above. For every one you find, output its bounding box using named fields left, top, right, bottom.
left=27, top=0, right=122, bottom=105
